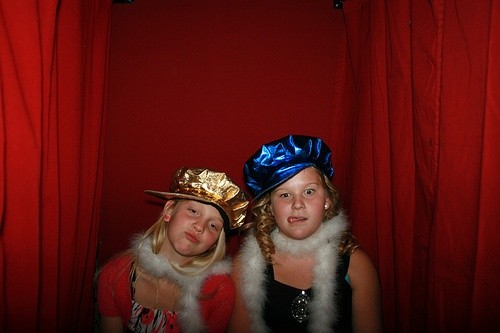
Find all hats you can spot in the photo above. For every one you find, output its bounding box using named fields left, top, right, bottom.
left=144, top=167, right=249, bottom=230
left=244, top=134, right=334, bottom=205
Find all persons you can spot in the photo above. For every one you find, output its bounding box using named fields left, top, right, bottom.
left=94, top=167, right=249, bottom=333
left=229, top=134, right=381, bottom=333
